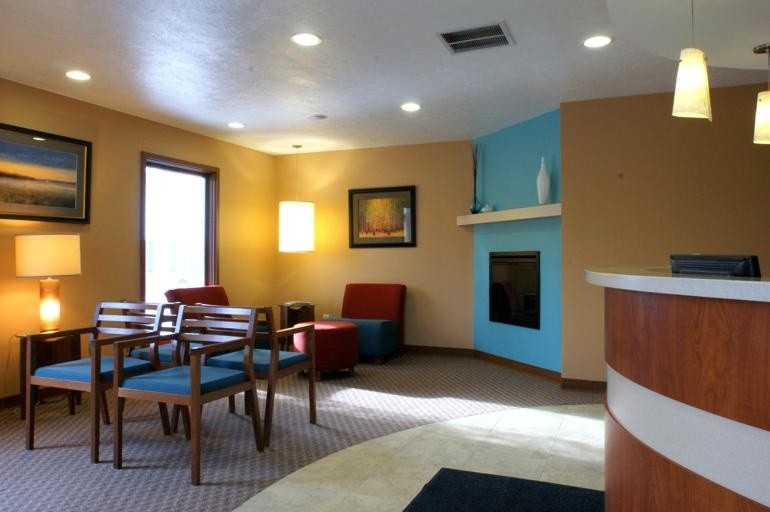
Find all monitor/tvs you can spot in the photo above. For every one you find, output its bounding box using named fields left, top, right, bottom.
left=670, top=254, right=761, bottom=277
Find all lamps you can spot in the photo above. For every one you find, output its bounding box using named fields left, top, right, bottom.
left=14, top=233, right=82, bottom=334
left=753, top=43, right=770, bottom=145
left=671, top=0, right=714, bottom=122
left=278, top=145, right=315, bottom=254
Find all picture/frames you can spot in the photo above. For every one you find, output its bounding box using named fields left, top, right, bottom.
left=0, top=122, right=91, bottom=224
left=348, top=186, right=417, bottom=248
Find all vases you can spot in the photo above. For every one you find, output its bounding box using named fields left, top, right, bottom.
left=537, top=155, right=549, bottom=206
left=470, top=191, right=480, bottom=214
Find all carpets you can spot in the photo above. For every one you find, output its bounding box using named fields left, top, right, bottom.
left=399, top=467, right=605, bottom=512
left=229, top=404, right=607, bottom=512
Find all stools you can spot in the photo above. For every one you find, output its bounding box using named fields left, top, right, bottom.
left=291, top=321, right=358, bottom=378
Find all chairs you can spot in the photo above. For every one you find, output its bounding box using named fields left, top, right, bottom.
left=118, top=294, right=207, bottom=435
left=321, top=283, right=406, bottom=363
left=25, top=301, right=172, bottom=464
left=112, top=305, right=266, bottom=484
left=196, top=301, right=319, bottom=446
